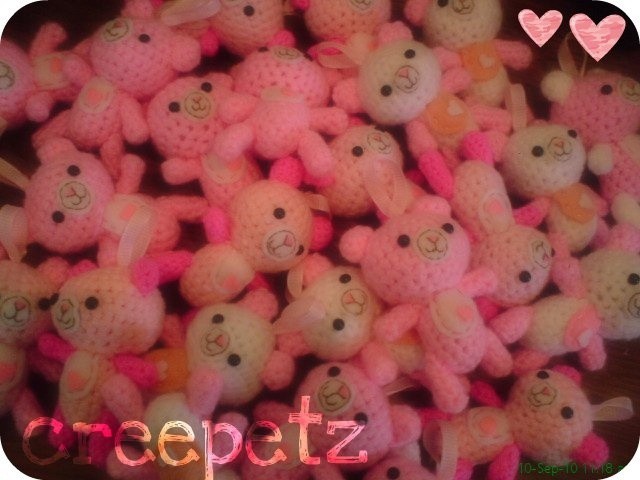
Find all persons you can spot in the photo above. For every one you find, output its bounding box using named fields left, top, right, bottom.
left=1, top=0, right=638, bottom=480
